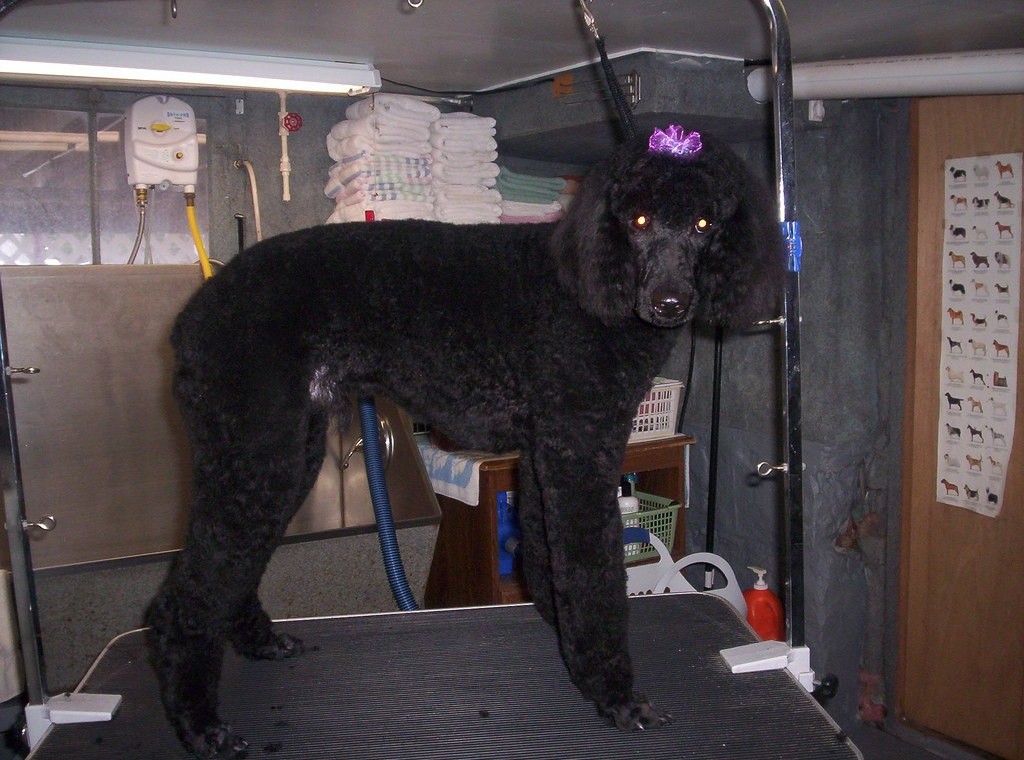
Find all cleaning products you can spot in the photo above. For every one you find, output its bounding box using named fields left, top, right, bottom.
left=741, top=565, right=784, bottom=641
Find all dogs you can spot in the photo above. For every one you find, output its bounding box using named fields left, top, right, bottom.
left=144, top=122, right=779, bottom=759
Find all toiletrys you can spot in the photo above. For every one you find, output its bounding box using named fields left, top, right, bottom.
left=615, top=472, right=640, bottom=554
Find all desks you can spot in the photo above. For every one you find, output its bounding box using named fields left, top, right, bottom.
left=28, top=593, right=861, bottom=760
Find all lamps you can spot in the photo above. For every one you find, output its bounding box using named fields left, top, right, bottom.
left=0, top=36, right=382, bottom=98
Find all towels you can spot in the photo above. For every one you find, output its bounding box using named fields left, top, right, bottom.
left=415, top=442, right=518, bottom=507
left=322, top=94, right=582, bottom=223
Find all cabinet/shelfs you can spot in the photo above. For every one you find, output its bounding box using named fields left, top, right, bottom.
left=412, top=432, right=698, bottom=609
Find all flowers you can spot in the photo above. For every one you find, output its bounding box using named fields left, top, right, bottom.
left=648, top=124, right=703, bottom=158
